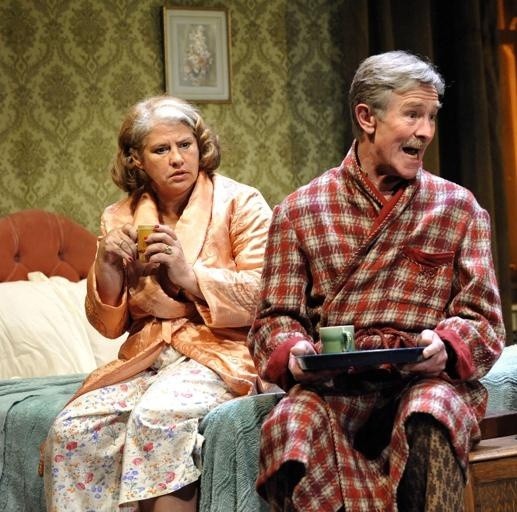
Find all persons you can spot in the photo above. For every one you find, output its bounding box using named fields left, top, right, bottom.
left=247, top=50, right=509, bottom=512
left=35, top=96, right=274, bottom=512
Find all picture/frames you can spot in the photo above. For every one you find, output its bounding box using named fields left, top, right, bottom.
left=161, top=5, right=233, bottom=106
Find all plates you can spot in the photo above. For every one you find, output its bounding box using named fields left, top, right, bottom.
left=297, top=347, right=429, bottom=370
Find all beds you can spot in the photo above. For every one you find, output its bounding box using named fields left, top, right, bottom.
left=0, top=207, right=515, bottom=512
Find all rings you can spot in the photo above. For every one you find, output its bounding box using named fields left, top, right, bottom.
left=163, top=244, right=171, bottom=253
left=119, top=240, right=124, bottom=246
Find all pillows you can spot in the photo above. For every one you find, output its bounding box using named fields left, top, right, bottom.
left=0, top=270, right=129, bottom=377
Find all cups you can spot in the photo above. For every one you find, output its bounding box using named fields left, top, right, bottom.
left=318, top=324, right=357, bottom=354
left=136, top=223, right=161, bottom=263
